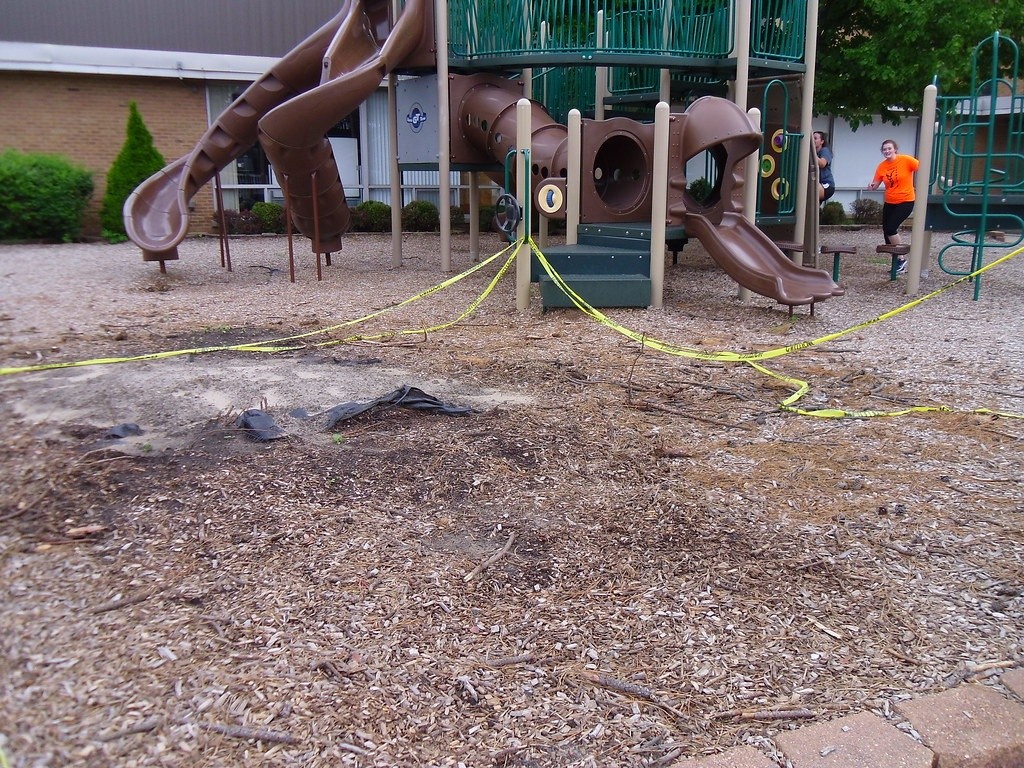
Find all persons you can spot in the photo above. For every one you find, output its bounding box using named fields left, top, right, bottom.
left=810, top=131, right=835, bottom=205
left=867, top=140, right=919, bottom=273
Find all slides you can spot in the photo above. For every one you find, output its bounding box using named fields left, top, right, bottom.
left=122, top=0, right=350, bottom=261
left=256, top=0, right=435, bottom=254
left=684, top=212, right=845, bottom=306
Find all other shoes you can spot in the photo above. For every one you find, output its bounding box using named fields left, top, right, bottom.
left=896, top=259, right=907, bottom=271
left=889, top=267, right=908, bottom=275
